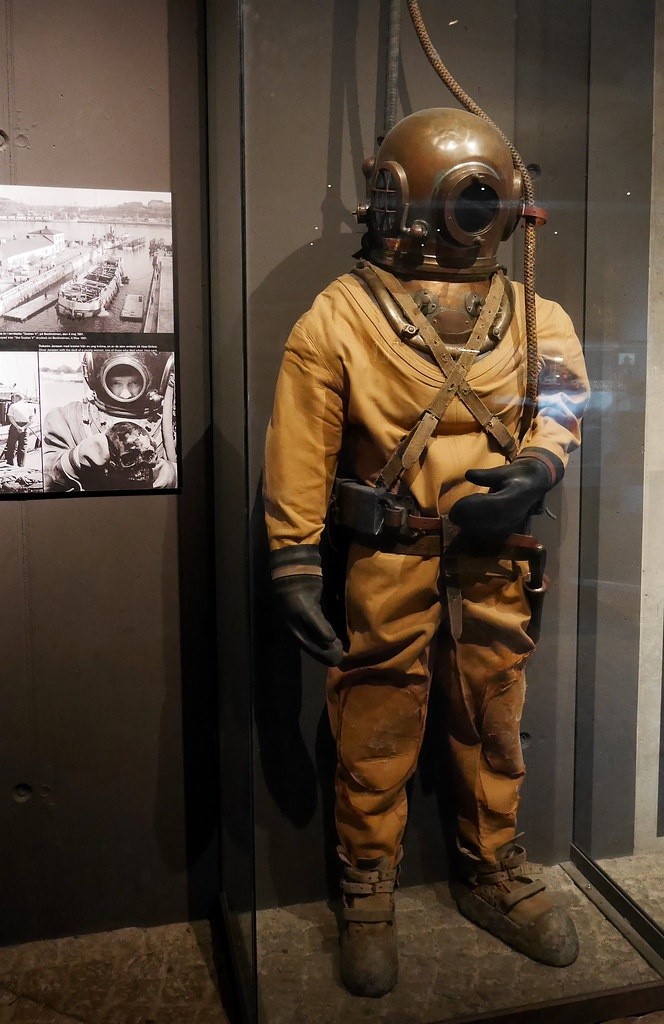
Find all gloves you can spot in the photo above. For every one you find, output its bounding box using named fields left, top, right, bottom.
left=59, top=432, right=110, bottom=480
left=448, top=447, right=564, bottom=530
left=267, top=544, right=343, bottom=667
left=151, top=458, right=176, bottom=489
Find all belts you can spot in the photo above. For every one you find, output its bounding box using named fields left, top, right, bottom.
left=348, top=507, right=538, bottom=640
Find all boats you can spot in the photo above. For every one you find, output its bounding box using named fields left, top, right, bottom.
left=56, top=252, right=126, bottom=320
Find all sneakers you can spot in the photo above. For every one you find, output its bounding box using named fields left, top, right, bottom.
left=452, top=831, right=579, bottom=966
left=334, top=845, right=405, bottom=999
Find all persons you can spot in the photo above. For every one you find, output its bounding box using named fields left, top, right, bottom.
left=0, top=226, right=179, bottom=492
left=262, top=110, right=594, bottom=989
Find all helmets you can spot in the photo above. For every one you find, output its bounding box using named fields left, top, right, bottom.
left=82, top=350, right=173, bottom=419
left=351, top=107, right=523, bottom=279
